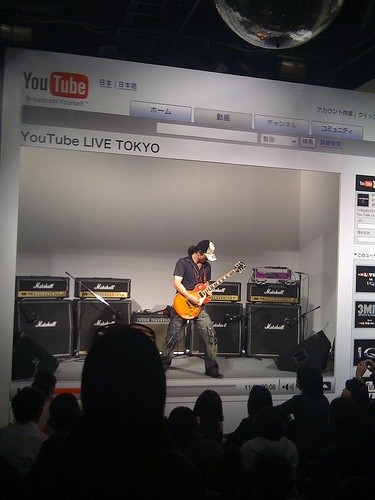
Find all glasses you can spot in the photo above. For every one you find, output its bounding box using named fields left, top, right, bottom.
left=92, top=323, right=156, bottom=344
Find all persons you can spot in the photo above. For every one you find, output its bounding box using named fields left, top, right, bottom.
left=160, top=240, right=224, bottom=379
left=25, top=323, right=210, bottom=500
left=0, top=359, right=375, bottom=500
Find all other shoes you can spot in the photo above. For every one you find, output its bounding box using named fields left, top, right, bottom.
left=205, top=371, right=224, bottom=378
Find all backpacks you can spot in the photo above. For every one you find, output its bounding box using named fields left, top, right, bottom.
left=248, top=439, right=294, bottom=500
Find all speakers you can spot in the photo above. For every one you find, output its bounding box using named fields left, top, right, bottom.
left=189, top=302, right=243, bottom=355
left=274, top=329, right=330, bottom=372
left=18, top=298, right=74, bottom=360
left=76, top=300, right=132, bottom=357
left=246, top=303, right=302, bottom=359
left=136, top=322, right=184, bottom=354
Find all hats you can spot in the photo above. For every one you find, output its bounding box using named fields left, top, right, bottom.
left=197, top=240, right=217, bottom=262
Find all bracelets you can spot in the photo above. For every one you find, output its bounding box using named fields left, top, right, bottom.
left=356, top=375, right=363, bottom=381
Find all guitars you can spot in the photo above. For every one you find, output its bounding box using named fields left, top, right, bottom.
left=174, top=261, right=246, bottom=319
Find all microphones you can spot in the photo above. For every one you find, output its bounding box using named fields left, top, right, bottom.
left=224, top=314, right=232, bottom=320
left=112, top=312, right=120, bottom=319
left=284, top=317, right=290, bottom=324
